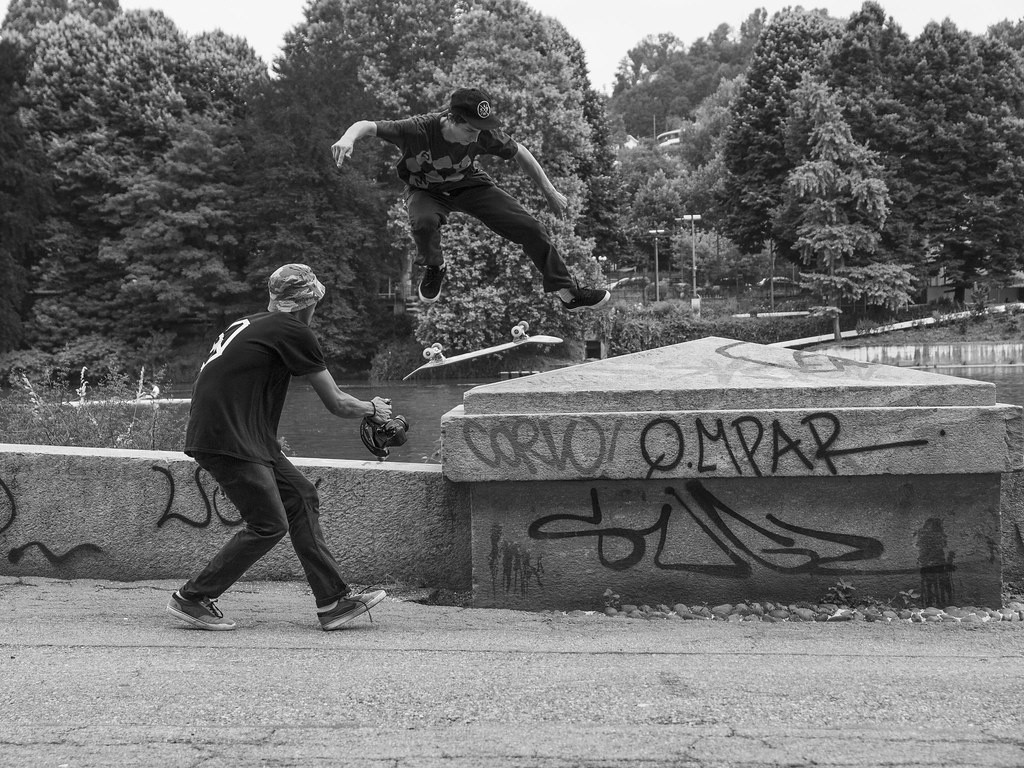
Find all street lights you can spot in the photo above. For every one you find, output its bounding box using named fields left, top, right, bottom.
left=683, top=212, right=704, bottom=299
left=648, top=229, right=664, bottom=303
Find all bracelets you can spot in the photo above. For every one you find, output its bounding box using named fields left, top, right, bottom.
left=368, top=401, right=376, bottom=418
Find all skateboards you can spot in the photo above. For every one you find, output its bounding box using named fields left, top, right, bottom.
left=403, top=321, right=563, bottom=381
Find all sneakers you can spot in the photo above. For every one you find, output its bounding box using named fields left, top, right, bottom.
left=166, top=591, right=237, bottom=630
left=560, top=287, right=611, bottom=312
left=418, top=265, right=447, bottom=303
left=317, top=589, right=387, bottom=630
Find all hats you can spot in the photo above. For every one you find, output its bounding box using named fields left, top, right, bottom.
left=450, top=87, right=502, bottom=129
left=267, top=263, right=326, bottom=313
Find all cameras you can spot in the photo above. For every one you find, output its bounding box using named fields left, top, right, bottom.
left=373, top=415, right=409, bottom=450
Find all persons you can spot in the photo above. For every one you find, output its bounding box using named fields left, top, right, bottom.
left=332, top=88, right=610, bottom=311
left=167, top=264, right=393, bottom=629
